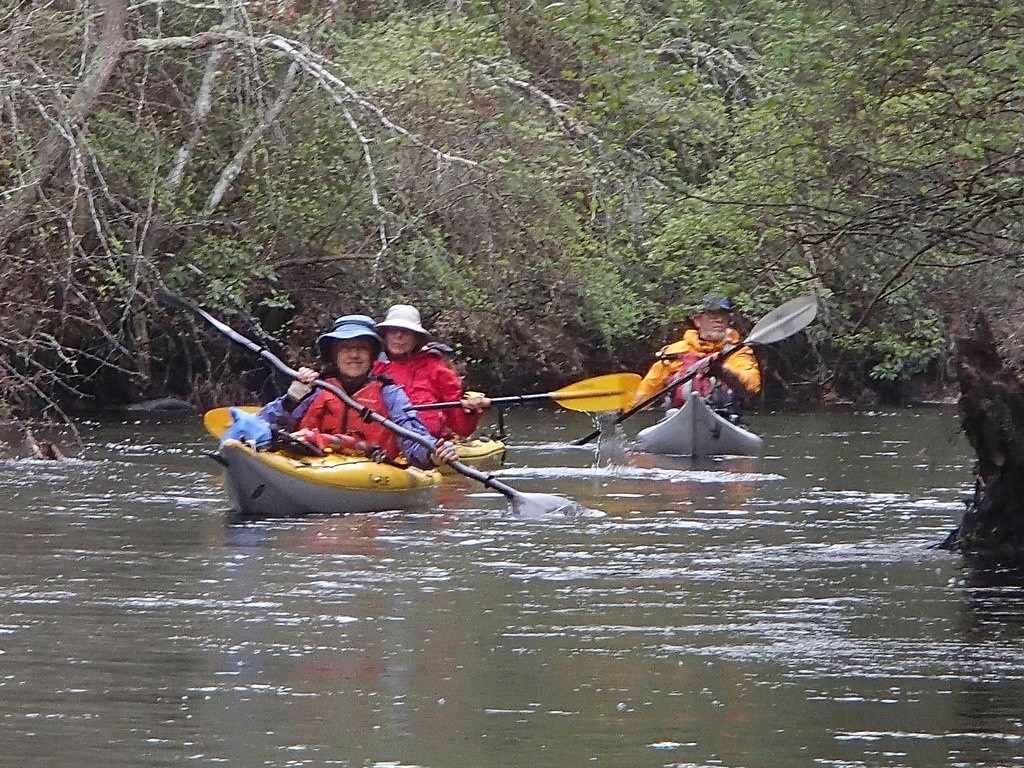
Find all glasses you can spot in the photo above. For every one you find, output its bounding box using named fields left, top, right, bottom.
left=335, top=342, right=372, bottom=353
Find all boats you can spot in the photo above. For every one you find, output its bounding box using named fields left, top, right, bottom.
left=634, top=388, right=765, bottom=459
left=437, top=402, right=509, bottom=478
left=198, top=406, right=445, bottom=518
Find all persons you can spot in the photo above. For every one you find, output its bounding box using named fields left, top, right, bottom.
left=253, top=314, right=458, bottom=468
left=374, top=304, right=483, bottom=442
left=614, top=294, right=762, bottom=433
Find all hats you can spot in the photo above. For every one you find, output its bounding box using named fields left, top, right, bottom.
left=376, top=304, right=432, bottom=336
left=316, top=315, right=385, bottom=357
left=691, top=293, right=736, bottom=313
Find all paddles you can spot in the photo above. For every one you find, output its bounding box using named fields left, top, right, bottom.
left=570, top=295, right=819, bottom=447
left=201, top=372, right=642, bottom=443
left=160, top=293, right=584, bottom=523
